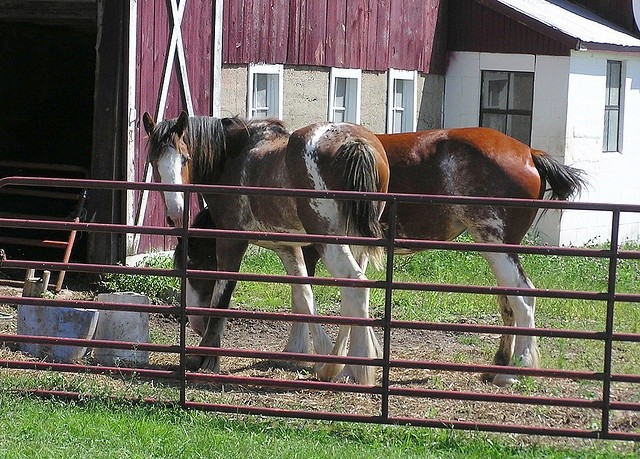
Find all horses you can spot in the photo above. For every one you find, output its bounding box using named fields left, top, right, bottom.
left=173, top=127, right=589, bottom=388
left=141, top=112, right=391, bottom=385
